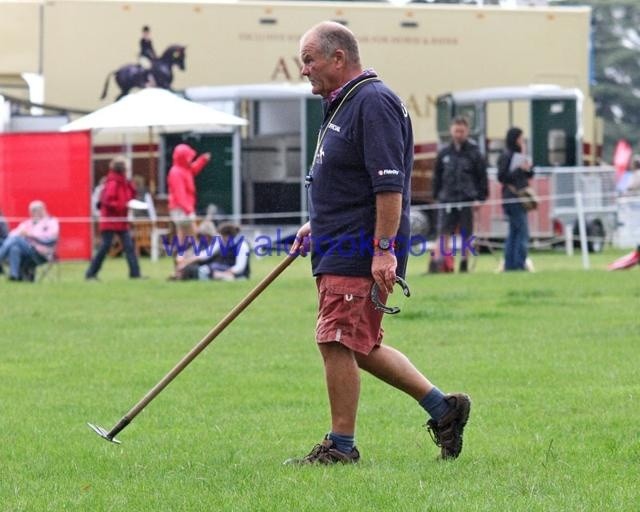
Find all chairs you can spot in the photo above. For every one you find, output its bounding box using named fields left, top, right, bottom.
left=36, top=251, right=61, bottom=283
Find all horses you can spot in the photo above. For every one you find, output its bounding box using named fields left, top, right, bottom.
left=100, top=45, right=186, bottom=102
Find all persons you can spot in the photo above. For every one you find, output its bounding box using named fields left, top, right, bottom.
left=625, top=156, right=640, bottom=199
left=167, top=224, right=251, bottom=281
left=0, top=200, right=60, bottom=282
left=496, top=127, right=538, bottom=274
left=83, top=156, right=155, bottom=281
left=279, top=20, right=472, bottom=470
left=431, top=115, right=490, bottom=274
left=138, top=25, right=158, bottom=88
left=173, top=217, right=219, bottom=279
left=165, top=143, right=213, bottom=282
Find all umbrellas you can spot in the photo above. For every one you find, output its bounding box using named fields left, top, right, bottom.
left=58, top=81, right=250, bottom=202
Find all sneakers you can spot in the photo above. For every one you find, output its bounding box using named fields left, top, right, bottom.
left=426, top=393, right=471, bottom=466
left=283, top=434, right=360, bottom=468
left=461, top=260, right=467, bottom=271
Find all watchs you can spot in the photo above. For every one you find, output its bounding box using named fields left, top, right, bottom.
left=374, top=236, right=395, bottom=251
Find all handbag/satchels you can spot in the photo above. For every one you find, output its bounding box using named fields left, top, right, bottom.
left=430, top=255, right=454, bottom=273
left=517, top=186, right=539, bottom=210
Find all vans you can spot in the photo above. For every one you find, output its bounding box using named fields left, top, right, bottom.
left=553, top=167, right=619, bottom=251
